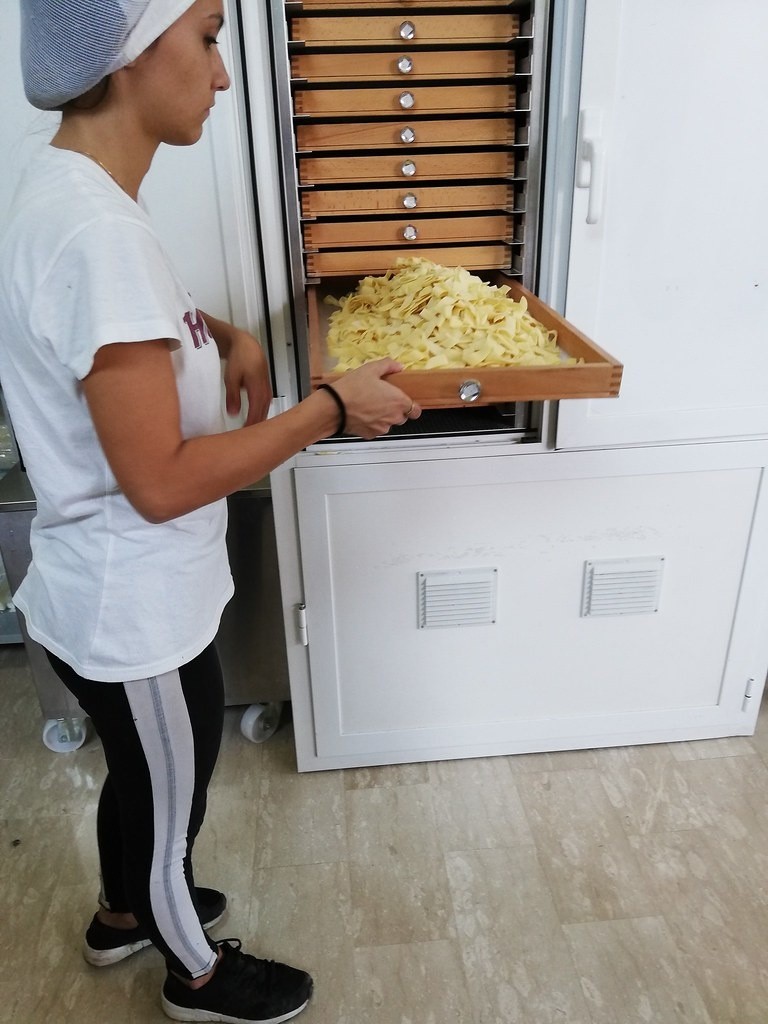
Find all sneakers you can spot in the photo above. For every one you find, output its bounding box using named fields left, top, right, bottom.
left=82, top=887, right=227, bottom=966
left=161, top=938, right=314, bottom=1024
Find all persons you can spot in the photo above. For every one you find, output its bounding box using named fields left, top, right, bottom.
left=0, top=0, right=424, bottom=1023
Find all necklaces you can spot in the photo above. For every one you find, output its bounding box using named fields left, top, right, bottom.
left=53, top=144, right=130, bottom=198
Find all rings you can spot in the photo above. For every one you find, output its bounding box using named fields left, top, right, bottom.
left=404, top=399, right=416, bottom=417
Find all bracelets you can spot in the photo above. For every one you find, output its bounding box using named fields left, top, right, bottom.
left=318, top=383, right=347, bottom=439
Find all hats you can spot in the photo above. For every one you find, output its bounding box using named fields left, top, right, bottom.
left=20, top=0, right=198, bottom=110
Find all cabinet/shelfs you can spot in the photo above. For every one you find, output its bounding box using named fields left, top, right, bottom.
left=204, top=0, right=768, bottom=776
